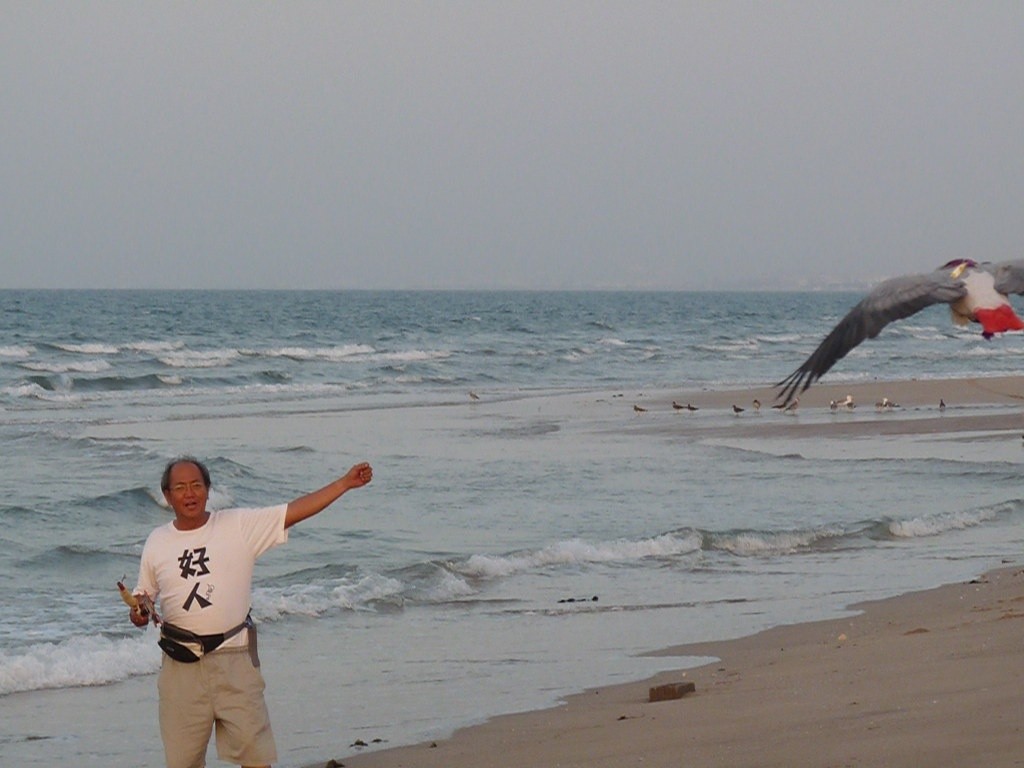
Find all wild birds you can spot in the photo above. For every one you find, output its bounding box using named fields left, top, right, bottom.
left=784, top=397, right=800, bottom=414
left=769, top=259, right=1023, bottom=409
left=752, top=399, right=760, bottom=408
left=873, top=397, right=902, bottom=411
left=672, top=401, right=686, bottom=412
left=731, top=405, right=746, bottom=415
left=634, top=405, right=646, bottom=414
left=771, top=405, right=787, bottom=412
left=684, top=402, right=698, bottom=412
left=830, top=394, right=857, bottom=412
left=939, top=398, right=947, bottom=412
left=469, top=390, right=478, bottom=401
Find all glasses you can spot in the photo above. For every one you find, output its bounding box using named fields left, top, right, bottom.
left=169, top=481, right=205, bottom=495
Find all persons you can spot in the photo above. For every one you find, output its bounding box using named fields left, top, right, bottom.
left=130, top=457, right=373, bottom=768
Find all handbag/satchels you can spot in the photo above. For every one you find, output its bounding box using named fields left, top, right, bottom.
left=157, top=616, right=262, bottom=670
